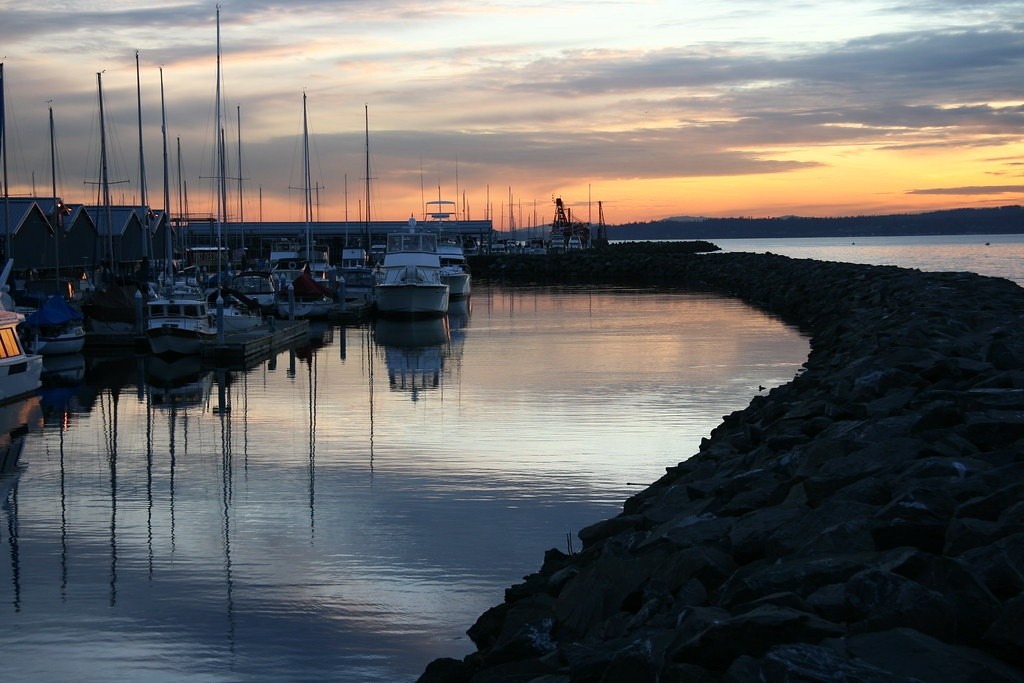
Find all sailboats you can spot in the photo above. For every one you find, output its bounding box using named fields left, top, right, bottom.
left=79, top=72, right=147, bottom=345
left=147, top=10, right=262, bottom=352
left=0, top=62, right=43, bottom=407
left=479, top=188, right=584, bottom=250
left=24, top=108, right=84, bottom=353
left=0, top=328, right=377, bottom=670
left=89, top=51, right=374, bottom=312
left=275, top=93, right=344, bottom=320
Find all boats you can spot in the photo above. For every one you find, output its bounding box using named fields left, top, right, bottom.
left=374, top=214, right=450, bottom=316
left=423, top=201, right=471, bottom=298
left=374, top=314, right=452, bottom=400
left=449, top=296, right=472, bottom=369
